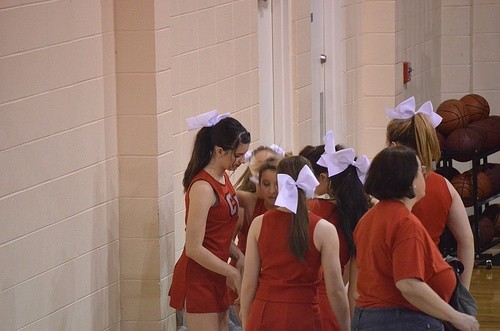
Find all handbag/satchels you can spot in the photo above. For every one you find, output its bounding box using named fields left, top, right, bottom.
left=448, top=257, right=479, bottom=331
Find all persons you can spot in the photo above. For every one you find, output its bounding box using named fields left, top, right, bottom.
left=350, top=145, right=483, bottom=331
left=381, top=98, right=477, bottom=290
left=231, top=145, right=280, bottom=193
left=227, top=157, right=284, bottom=253
left=304, top=145, right=373, bottom=285
left=166, top=111, right=253, bottom=330
left=239, top=153, right=352, bottom=330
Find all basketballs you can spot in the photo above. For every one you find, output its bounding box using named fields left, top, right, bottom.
left=429, top=92, right=500, bottom=252
